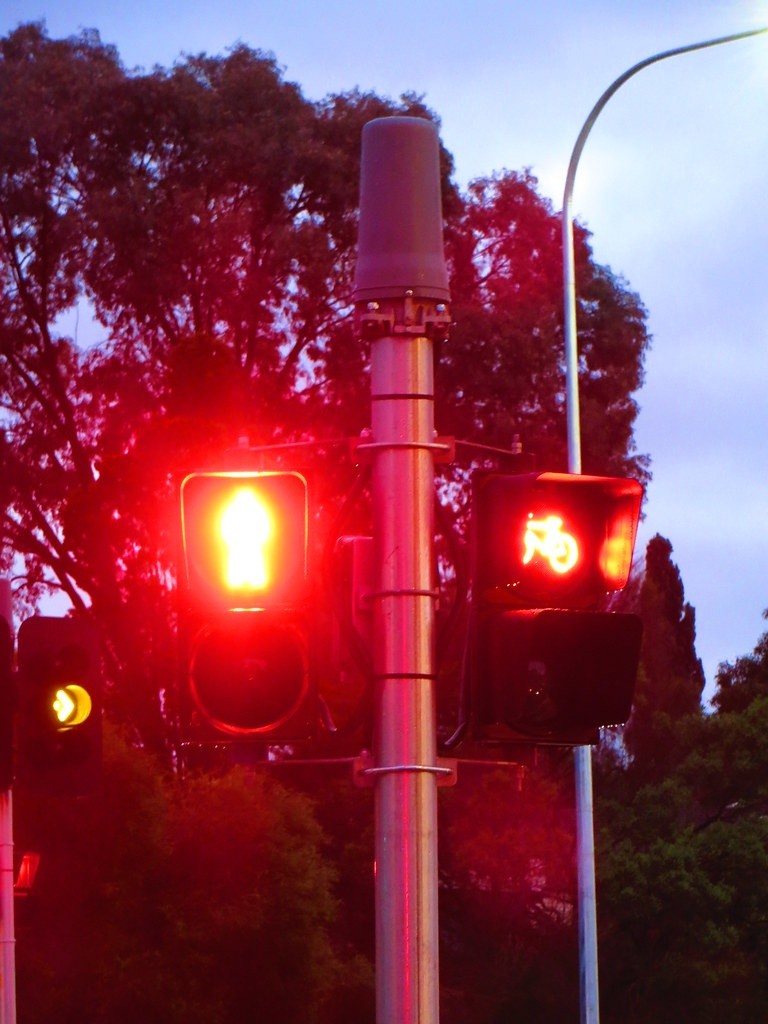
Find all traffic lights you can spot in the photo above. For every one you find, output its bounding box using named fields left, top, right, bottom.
left=489, top=608, right=639, bottom=742
left=170, top=469, right=327, bottom=736
left=17, top=615, right=93, bottom=792
left=479, top=470, right=643, bottom=613
